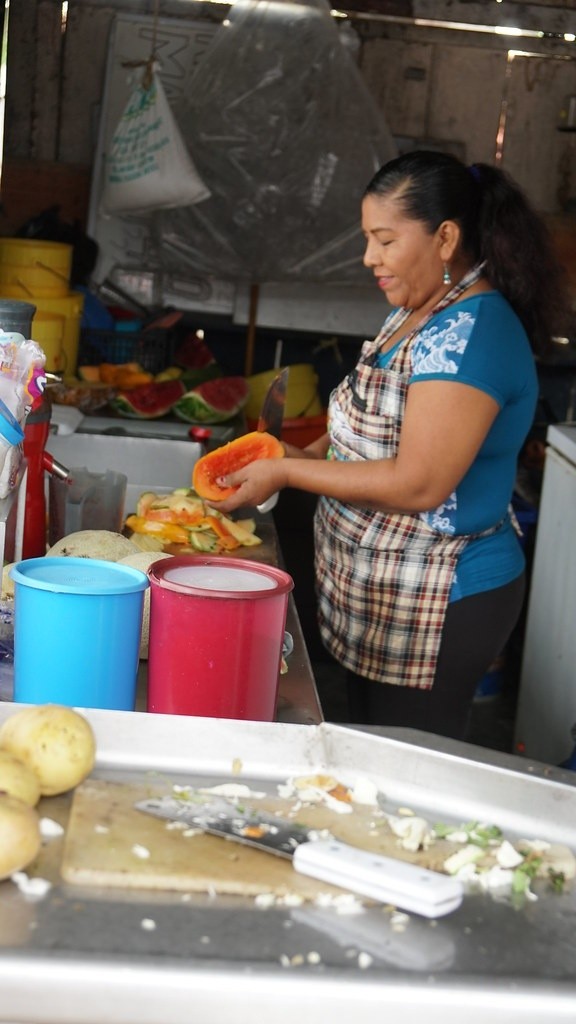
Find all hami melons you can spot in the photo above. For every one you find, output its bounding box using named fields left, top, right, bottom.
left=43, top=528, right=175, bottom=661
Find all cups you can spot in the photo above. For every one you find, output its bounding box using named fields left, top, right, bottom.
left=50, top=467, right=127, bottom=551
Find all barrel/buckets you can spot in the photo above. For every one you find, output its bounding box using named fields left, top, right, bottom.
left=0, top=237, right=83, bottom=382
left=146, top=551, right=295, bottom=723
left=8, top=555, right=151, bottom=715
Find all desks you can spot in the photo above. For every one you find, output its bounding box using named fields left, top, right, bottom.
left=0, top=473, right=327, bottom=731
left=0, top=701, right=576, bottom=1013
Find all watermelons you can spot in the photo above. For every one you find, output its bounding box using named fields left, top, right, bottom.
left=110, top=374, right=251, bottom=426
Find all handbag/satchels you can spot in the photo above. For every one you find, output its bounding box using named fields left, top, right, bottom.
left=97, top=62, right=212, bottom=219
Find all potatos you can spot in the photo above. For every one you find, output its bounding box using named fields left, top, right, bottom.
left=1, top=704, right=97, bottom=881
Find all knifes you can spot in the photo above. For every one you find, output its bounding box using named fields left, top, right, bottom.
left=253, top=366, right=288, bottom=515
left=281, top=903, right=464, bottom=971
left=137, top=799, right=466, bottom=920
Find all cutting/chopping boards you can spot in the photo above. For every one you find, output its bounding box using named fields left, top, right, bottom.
left=61, top=777, right=403, bottom=904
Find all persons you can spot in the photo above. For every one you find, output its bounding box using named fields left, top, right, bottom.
left=207, top=149, right=568, bottom=735
